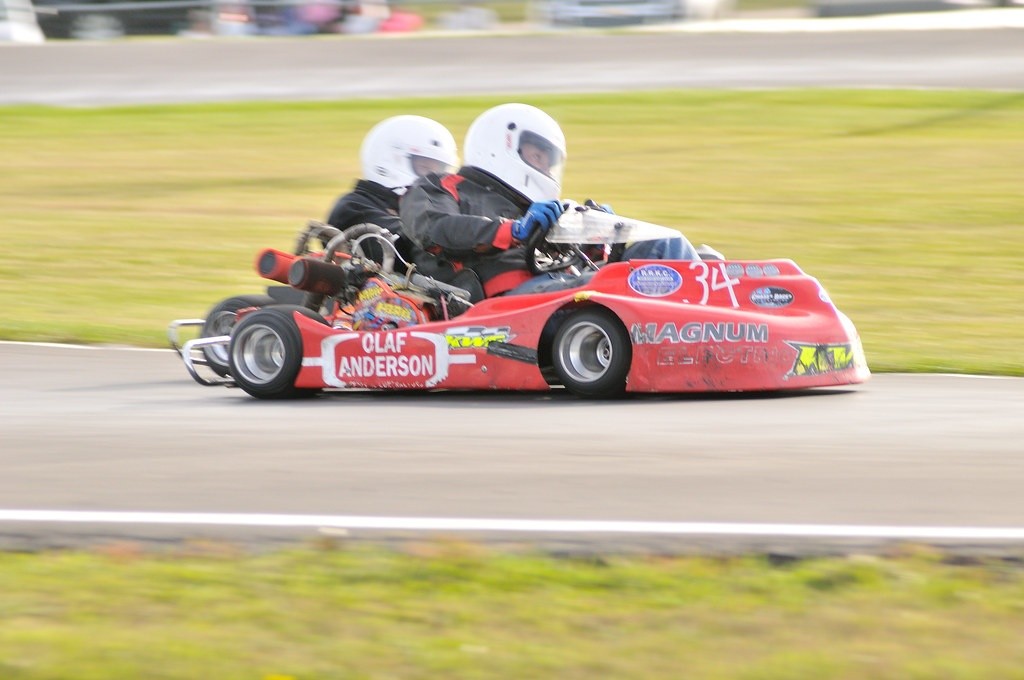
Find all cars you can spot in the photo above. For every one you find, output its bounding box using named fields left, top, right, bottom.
left=815, top=0, right=1023, bottom=17
left=548, top=0, right=686, bottom=30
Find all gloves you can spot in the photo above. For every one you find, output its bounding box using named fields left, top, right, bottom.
left=585, top=199, right=618, bottom=215
left=511, top=199, right=569, bottom=241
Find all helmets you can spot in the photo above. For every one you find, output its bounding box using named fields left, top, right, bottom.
left=359, top=114, right=459, bottom=196
left=463, top=103, right=567, bottom=204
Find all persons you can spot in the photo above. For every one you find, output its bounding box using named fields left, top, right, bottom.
left=399, top=102, right=725, bottom=299
left=320, top=115, right=459, bottom=275
